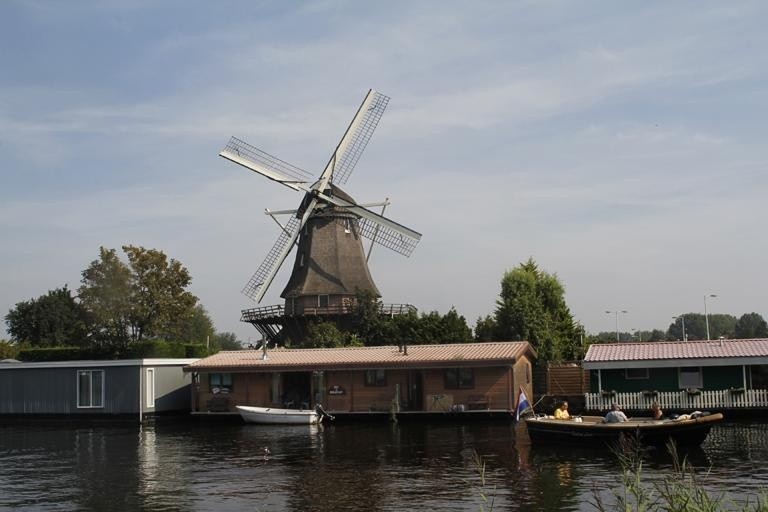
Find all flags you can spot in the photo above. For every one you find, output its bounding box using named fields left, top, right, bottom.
left=513, top=384, right=536, bottom=421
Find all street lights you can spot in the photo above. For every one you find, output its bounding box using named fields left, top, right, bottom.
left=606, top=310, right=629, bottom=342
left=631, top=327, right=641, bottom=342
left=703, top=292, right=717, bottom=339
left=672, top=315, right=685, bottom=341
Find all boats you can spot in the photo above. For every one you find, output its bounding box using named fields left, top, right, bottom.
left=235, top=424, right=325, bottom=441
left=528, top=439, right=713, bottom=473
left=523, top=408, right=724, bottom=443
left=236, top=403, right=338, bottom=424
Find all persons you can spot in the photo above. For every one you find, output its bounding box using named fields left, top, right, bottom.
left=650, top=402, right=663, bottom=419
left=602, top=403, right=633, bottom=423
left=554, top=400, right=575, bottom=420
